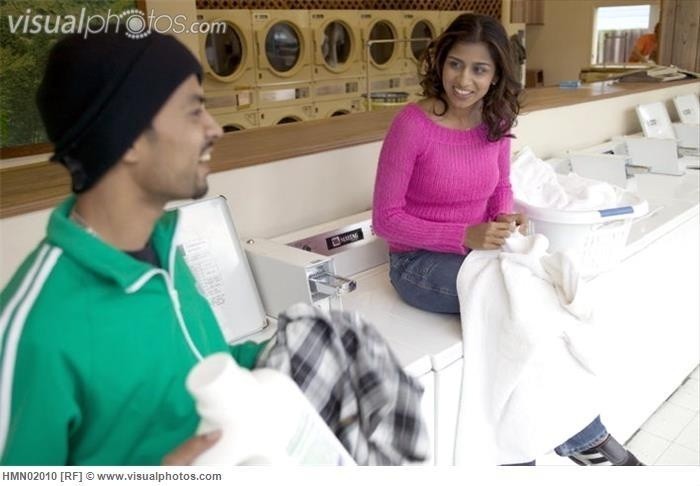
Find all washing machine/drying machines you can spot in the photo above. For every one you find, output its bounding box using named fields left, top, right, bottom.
left=196, top=10, right=473, bottom=134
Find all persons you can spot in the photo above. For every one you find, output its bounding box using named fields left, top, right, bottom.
left=2, top=27, right=272, bottom=466
left=628, top=24, right=661, bottom=62
left=369, top=14, right=641, bottom=467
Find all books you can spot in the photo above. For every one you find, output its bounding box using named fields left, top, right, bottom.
left=614, top=64, right=698, bottom=85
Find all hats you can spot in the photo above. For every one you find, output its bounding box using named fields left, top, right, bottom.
left=38, top=22, right=203, bottom=194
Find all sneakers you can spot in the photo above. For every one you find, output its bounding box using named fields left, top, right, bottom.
left=568, top=435, right=645, bottom=466
